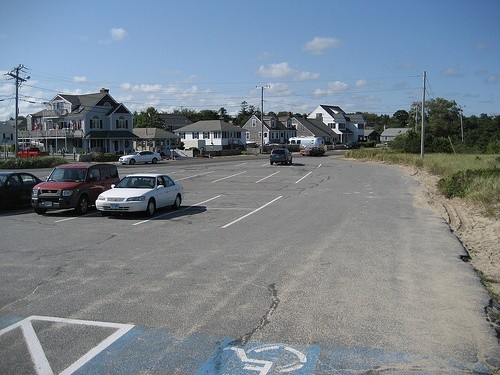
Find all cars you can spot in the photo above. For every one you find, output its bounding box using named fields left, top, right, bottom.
left=94, top=174, right=184, bottom=219
left=119, top=151, right=160, bottom=166
left=344, top=142, right=360, bottom=151
left=0, top=172, right=49, bottom=208
left=300, top=146, right=326, bottom=157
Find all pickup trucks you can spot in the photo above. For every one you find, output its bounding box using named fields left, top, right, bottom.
left=17, top=147, right=50, bottom=157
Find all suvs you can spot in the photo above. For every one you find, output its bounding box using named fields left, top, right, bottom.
left=31, top=161, right=120, bottom=217
left=270, top=148, right=293, bottom=166
